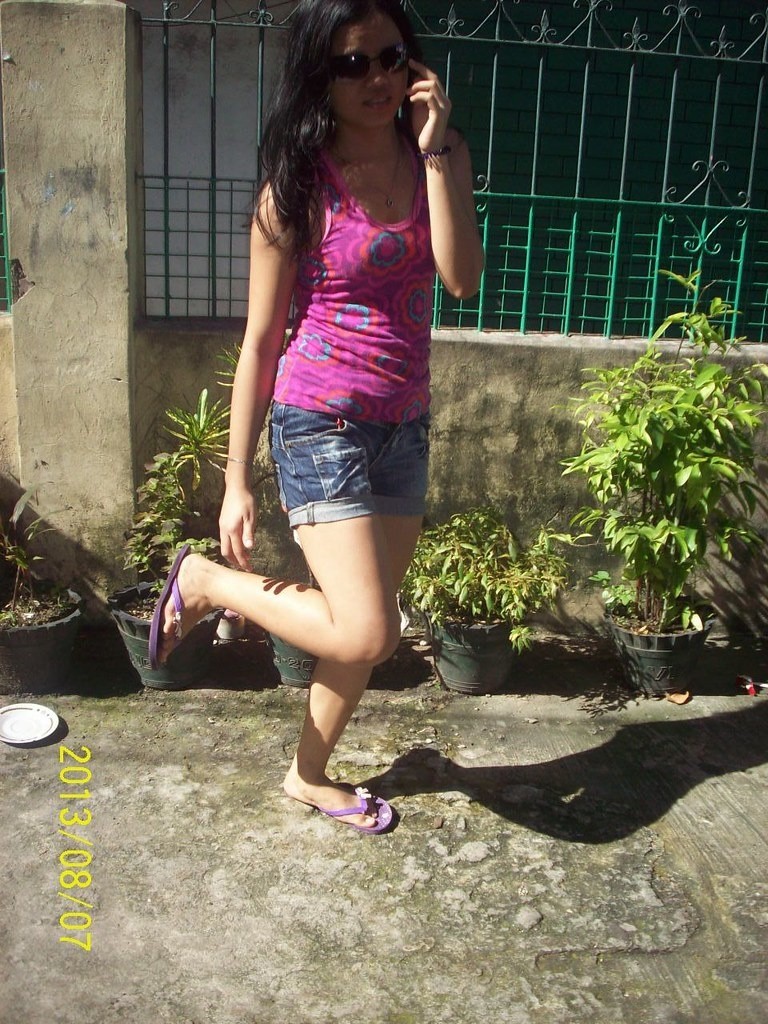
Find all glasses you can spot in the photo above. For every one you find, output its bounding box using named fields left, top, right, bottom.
left=328, top=39, right=414, bottom=80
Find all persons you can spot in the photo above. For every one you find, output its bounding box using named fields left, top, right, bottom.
left=148, top=0, right=487, bottom=835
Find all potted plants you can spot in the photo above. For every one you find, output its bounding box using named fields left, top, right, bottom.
left=0, top=480, right=88, bottom=690
left=214, top=337, right=347, bottom=689
left=394, top=505, right=576, bottom=696
left=106, top=386, right=237, bottom=694
left=551, top=266, right=768, bottom=696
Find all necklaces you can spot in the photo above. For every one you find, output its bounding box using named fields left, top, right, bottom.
left=357, top=146, right=401, bottom=208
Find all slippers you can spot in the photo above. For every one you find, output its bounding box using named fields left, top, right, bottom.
left=312, top=781, right=395, bottom=836
left=148, top=542, right=194, bottom=674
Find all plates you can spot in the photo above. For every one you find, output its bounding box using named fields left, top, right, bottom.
left=0, top=703, right=59, bottom=743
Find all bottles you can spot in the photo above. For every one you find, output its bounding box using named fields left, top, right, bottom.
left=601, top=588, right=617, bottom=605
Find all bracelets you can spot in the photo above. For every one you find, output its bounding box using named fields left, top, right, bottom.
left=417, top=145, right=452, bottom=159
left=227, top=456, right=252, bottom=468
